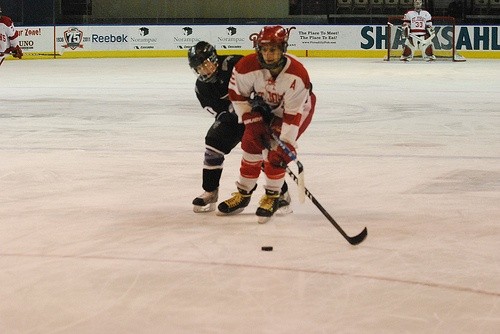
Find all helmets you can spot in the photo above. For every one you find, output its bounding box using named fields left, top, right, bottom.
left=256, top=24, right=288, bottom=50
left=187, top=40, right=219, bottom=83
left=414, top=0, right=422, bottom=8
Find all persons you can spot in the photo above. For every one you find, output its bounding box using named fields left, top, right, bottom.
left=0, top=15, right=23, bottom=66
left=400, top=0, right=437, bottom=62
left=187, top=41, right=245, bottom=213
left=218, top=25, right=316, bottom=224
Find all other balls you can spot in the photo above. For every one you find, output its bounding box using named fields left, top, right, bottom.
left=262, top=246, right=273, bottom=251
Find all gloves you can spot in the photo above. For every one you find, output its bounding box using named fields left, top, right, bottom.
left=268, top=142, right=297, bottom=168
left=242, top=111, right=270, bottom=149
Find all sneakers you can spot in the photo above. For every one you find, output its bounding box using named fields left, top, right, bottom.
left=192, top=187, right=218, bottom=213
left=216, top=181, right=258, bottom=216
left=256, top=185, right=281, bottom=224
left=275, top=181, right=294, bottom=217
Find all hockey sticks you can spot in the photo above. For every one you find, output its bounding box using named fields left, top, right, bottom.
left=0, top=45, right=65, bottom=57
left=263, top=135, right=369, bottom=246
left=272, top=132, right=305, bottom=205
left=387, top=22, right=436, bottom=44
